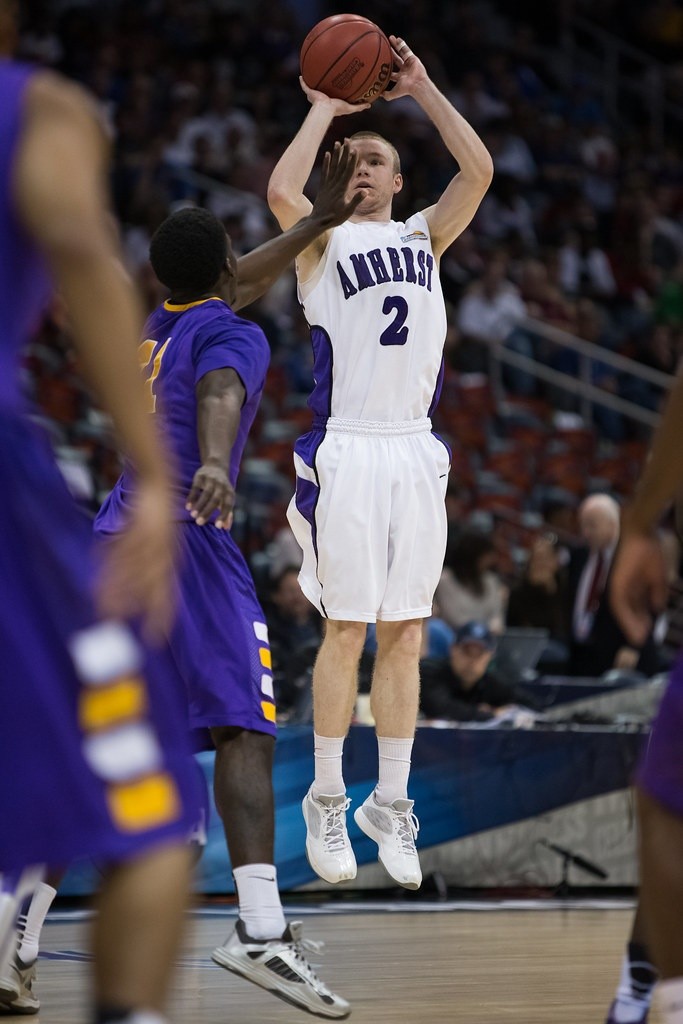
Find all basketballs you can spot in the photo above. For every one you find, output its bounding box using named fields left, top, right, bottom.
left=300, top=14, right=393, bottom=107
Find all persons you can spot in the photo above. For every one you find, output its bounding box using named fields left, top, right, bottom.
left=3, top=0, right=683, bottom=512
left=267, top=34, right=495, bottom=890
left=234, top=490, right=683, bottom=727
left=607, top=363, right=683, bottom=1024
left=0, top=10, right=207, bottom=1021
left=1, top=138, right=365, bottom=1022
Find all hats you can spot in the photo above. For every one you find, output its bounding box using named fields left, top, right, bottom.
left=456, top=622, right=495, bottom=647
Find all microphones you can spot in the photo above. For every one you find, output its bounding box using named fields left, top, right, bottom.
left=547, top=844, right=609, bottom=879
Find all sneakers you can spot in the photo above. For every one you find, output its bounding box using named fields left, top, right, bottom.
left=0, top=950, right=40, bottom=1013
left=354, top=791, right=422, bottom=891
left=302, top=781, right=358, bottom=883
left=211, top=919, right=350, bottom=1020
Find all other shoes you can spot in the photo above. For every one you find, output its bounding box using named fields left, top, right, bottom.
left=606, top=946, right=658, bottom=1024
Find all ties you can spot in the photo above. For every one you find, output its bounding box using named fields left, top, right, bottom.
left=586, top=552, right=604, bottom=611
